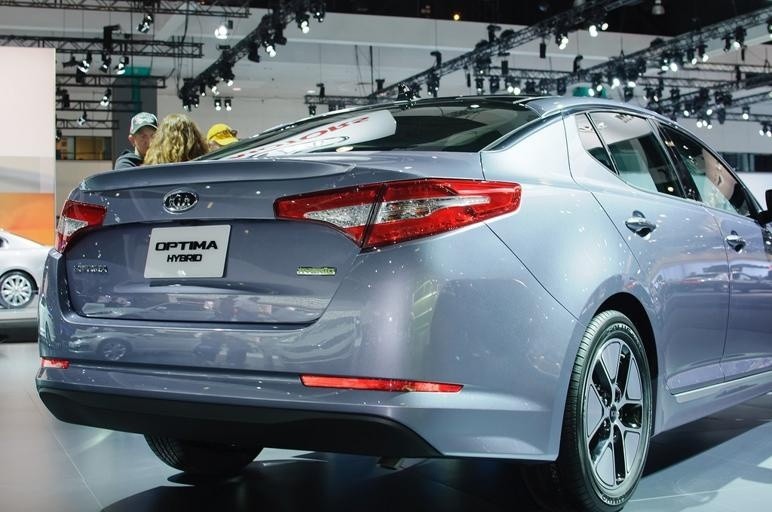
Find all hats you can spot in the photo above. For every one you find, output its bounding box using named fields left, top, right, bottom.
left=129, top=112, right=158, bottom=136
left=207, top=123, right=239, bottom=146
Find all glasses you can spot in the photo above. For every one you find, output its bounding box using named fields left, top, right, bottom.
left=208, top=128, right=237, bottom=140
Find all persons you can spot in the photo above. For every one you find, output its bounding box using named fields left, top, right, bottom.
left=112, top=108, right=240, bottom=169
left=55, top=128, right=66, bottom=157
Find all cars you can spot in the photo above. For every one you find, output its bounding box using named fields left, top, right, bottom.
left=64, top=298, right=373, bottom=375
left=35, top=91, right=771, bottom=512
left=0, top=226, right=52, bottom=309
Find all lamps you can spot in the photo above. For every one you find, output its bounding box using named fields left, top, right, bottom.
left=660, top=168, right=675, bottom=194
left=56, top=0, right=326, bottom=143
